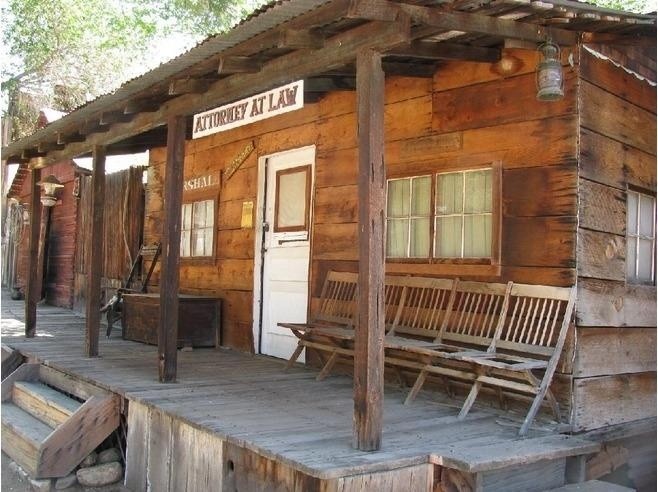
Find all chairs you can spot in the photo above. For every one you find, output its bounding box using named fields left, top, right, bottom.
left=103, top=241, right=159, bottom=337
left=306, top=274, right=410, bottom=338
left=384, top=276, right=459, bottom=403
left=401, top=279, right=513, bottom=420
left=276, top=268, right=358, bottom=381
left=451, top=282, right=575, bottom=436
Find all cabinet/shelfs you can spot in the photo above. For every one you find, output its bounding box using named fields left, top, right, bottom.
left=121, top=293, right=221, bottom=352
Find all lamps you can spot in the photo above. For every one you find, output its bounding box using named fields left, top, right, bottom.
left=35, top=166, right=65, bottom=207
left=532, top=43, right=565, bottom=101
